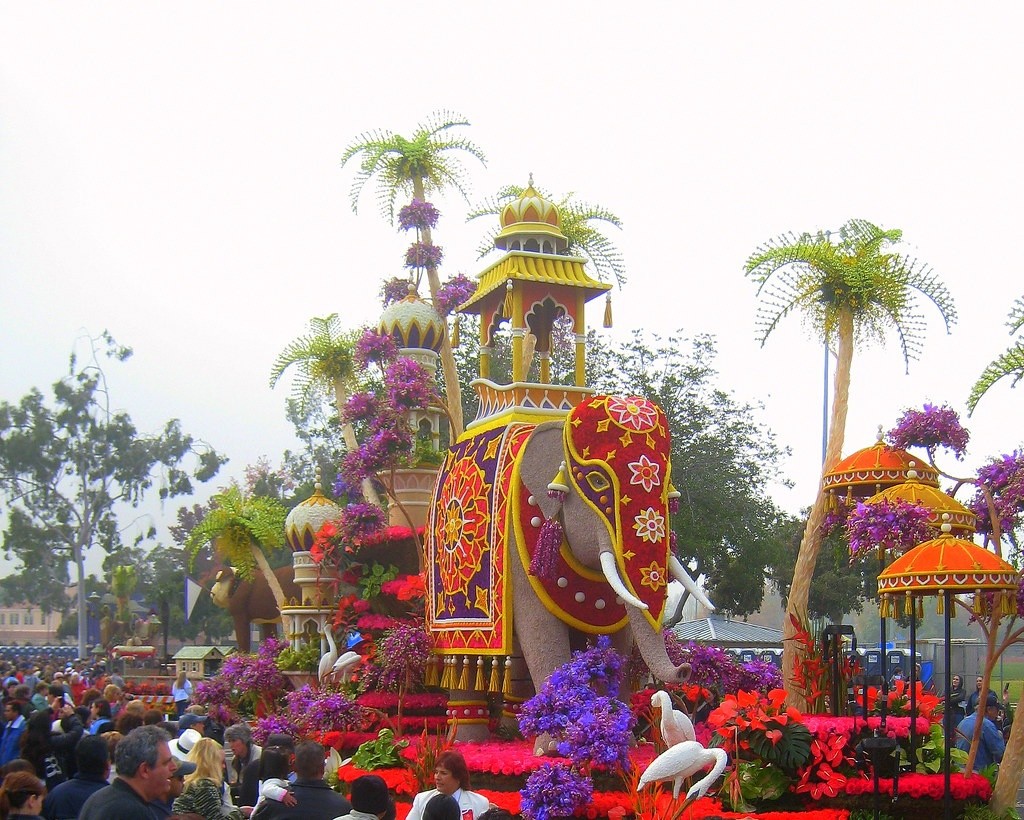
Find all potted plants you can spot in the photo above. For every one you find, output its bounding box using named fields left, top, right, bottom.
left=277, top=637, right=320, bottom=689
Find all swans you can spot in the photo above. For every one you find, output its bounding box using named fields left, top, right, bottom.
left=636, top=690, right=727, bottom=819
left=318, top=619, right=361, bottom=692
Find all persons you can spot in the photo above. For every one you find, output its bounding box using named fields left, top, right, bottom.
left=0, top=652, right=397, bottom=820
left=890, top=663, right=921, bottom=690
left=405, top=750, right=517, bottom=819
left=942, top=675, right=965, bottom=737
left=172, top=672, right=192, bottom=716
left=956, top=676, right=1012, bottom=769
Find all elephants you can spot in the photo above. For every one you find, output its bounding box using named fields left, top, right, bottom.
left=425, top=398, right=716, bottom=747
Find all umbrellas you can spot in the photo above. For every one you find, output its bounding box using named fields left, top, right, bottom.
left=822, top=446, right=940, bottom=732
left=855, top=482, right=976, bottom=773
left=877, top=534, right=1019, bottom=808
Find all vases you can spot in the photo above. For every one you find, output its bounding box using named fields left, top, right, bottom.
left=377, top=468, right=438, bottom=526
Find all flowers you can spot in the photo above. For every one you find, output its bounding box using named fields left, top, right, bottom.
left=400, top=433, right=447, bottom=470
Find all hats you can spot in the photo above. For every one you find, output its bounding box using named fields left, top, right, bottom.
left=167, top=729, right=202, bottom=760
left=986, top=695, right=1005, bottom=710
left=171, top=755, right=197, bottom=777
left=423, top=793, right=460, bottom=820
left=268, top=734, right=295, bottom=753
left=351, top=774, right=388, bottom=813
left=179, top=714, right=208, bottom=729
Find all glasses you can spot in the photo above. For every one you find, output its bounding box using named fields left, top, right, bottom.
left=176, top=776, right=183, bottom=781
